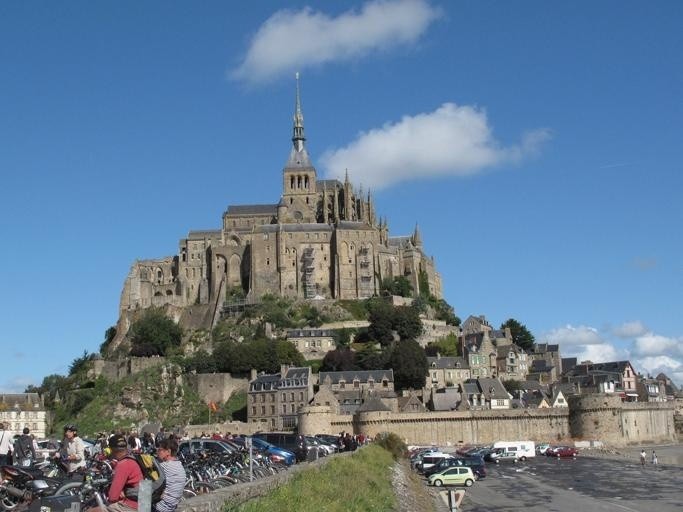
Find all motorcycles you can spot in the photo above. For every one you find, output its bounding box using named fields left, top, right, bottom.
left=0, top=455, right=103, bottom=512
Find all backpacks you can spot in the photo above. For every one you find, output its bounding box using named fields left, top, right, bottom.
left=118, top=453, right=166, bottom=504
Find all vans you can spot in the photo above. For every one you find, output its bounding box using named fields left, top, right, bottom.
left=425, top=455, right=486, bottom=481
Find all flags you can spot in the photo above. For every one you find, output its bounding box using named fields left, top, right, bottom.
left=207, top=398, right=216, bottom=414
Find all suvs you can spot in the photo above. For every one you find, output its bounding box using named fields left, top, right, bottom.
left=8, top=433, right=20, bottom=445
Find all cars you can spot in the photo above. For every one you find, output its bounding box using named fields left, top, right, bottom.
left=455, top=440, right=535, bottom=462
left=536, top=443, right=579, bottom=456
left=406, top=445, right=461, bottom=470
left=428, top=466, right=475, bottom=488
left=80, top=439, right=94, bottom=446
left=36, top=439, right=62, bottom=457
left=177, top=437, right=295, bottom=465
left=253, top=434, right=355, bottom=460
left=552, top=456, right=575, bottom=464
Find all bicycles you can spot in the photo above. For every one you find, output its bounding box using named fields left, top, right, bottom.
left=84, top=450, right=288, bottom=503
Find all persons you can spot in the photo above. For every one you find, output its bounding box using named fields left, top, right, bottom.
left=147, top=438, right=187, bottom=511
left=85, top=434, right=146, bottom=512
left=0, top=422, right=41, bottom=469
left=93, top=427, right=233, bottom=454
left=651, top=449, right=658, bottom=464
left=60, top=424, right=88, bottom=474
left=337, top=430, right=376, bottom=452
left=637, top=447, right=647, bottom=465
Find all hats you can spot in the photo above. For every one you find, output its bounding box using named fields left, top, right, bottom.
left=109, top=435, right=126, bottom=446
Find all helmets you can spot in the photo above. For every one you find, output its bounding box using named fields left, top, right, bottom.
left=63, top=424, right=77, bottom=431
